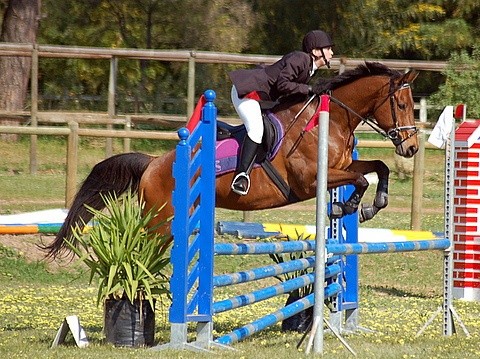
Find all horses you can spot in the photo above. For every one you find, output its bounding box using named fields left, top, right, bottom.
left=34, top=60, right=419, bottom=268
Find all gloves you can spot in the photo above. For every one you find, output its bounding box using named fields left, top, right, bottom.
left=308, top=84, right=323, bottom=94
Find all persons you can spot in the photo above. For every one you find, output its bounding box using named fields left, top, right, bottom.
left=228, top=30, right=335, bottom=191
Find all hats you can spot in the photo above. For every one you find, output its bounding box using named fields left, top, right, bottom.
left=302, top=29, right=335, bottom=53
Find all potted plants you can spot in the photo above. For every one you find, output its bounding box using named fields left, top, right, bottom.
left=262, top=227, right=316, bottom=332
left=62, top=187, right=174, bottom=348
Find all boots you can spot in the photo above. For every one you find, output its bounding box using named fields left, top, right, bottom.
left=234, top=134, right=261, bottom=191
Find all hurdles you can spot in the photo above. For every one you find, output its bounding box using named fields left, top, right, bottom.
left=150, top=88, right=371, bottom=355
left=303, top=95, right=471, bottom=358
